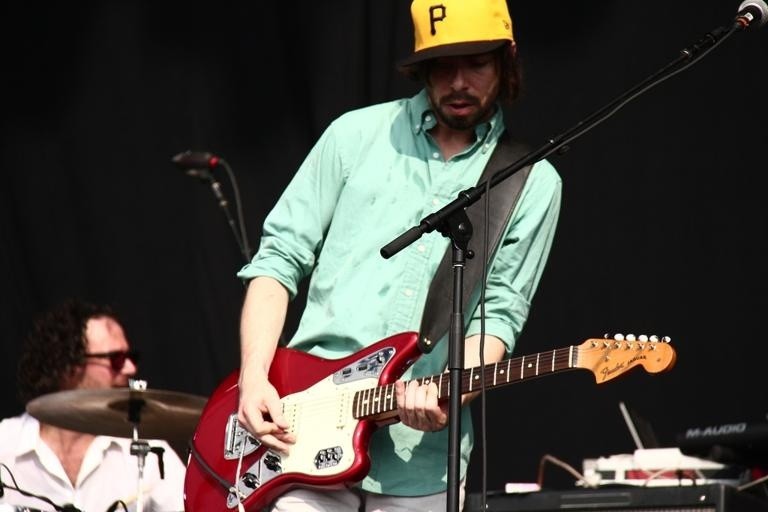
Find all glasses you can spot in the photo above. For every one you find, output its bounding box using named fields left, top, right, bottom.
left=83, top=350, right=137, bottom=373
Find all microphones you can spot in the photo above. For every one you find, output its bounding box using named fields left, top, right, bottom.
left=171, top=151, right=223, bottom=165
left=736, top=0, right=768, bottom=31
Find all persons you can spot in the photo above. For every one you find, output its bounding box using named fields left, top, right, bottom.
left=1, top=295, right=188, bottom=510
left=237, top=0, right=563, bottom=511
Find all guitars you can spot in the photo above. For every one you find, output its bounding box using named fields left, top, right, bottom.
left=183, top=332, right=676, bottom=512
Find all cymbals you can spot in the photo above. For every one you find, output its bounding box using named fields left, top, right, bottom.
left=25, top=389, right=208, bottom=438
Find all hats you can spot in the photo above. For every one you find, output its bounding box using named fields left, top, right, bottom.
left=398, top=0, right=516, bottom=67
